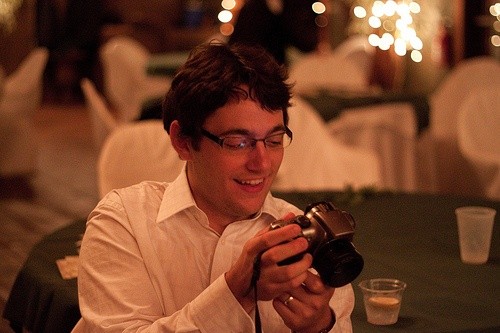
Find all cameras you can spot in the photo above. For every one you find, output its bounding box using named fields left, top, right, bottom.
left=268, top=201, right=364, bottom=287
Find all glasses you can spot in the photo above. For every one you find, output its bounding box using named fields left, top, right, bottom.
left=201, top=124, right=293, bottom=153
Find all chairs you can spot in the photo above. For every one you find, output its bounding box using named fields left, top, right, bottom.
left=81, top=38, right=500, bottom=197
left=0, top=49, right=49, bottom=198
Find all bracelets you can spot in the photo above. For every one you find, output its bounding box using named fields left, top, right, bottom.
left=319, top=310, right=335, bottom=333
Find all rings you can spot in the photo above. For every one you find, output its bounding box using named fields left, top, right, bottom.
left=283, top=296, right=293, bottom=306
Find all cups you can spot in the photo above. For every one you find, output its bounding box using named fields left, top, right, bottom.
left=359, top=279, right=405, bottom=325
left=456, top=207, right=495, bottom=263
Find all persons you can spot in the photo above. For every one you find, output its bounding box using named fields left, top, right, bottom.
left=37, top=0, right=322, bottom=103
left=70, top=39, right=356, bottom=333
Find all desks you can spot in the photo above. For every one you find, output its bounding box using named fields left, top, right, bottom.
left=275, top=188, right=500, bottom=333
left=3, top=218, right=87, bottom=333
